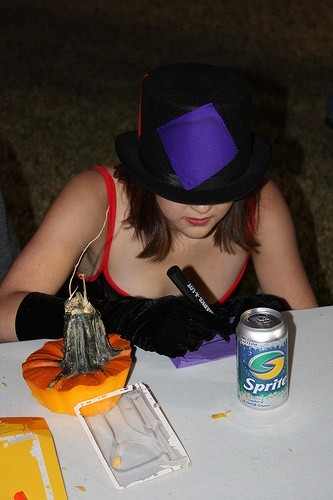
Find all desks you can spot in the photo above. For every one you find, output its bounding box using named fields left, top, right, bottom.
left=0, top=304, right=333, bottom=500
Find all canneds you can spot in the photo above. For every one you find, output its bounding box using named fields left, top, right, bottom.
left=234, top=306, right=289, bottom=411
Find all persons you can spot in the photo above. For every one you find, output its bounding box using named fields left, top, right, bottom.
left=0, top=52, right=320, bottom=355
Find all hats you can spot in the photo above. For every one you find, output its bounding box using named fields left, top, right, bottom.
left=114, top=60, right=273, bottom=205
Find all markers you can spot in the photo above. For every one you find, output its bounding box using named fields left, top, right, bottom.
left=167, top=263, right=232, bottom=343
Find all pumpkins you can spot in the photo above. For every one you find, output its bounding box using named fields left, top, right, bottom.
left=21, top=293, right=133, bottom=416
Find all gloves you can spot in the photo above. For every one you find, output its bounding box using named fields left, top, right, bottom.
left=216, top=295, right=290, bottom=334
left=15, top=292, right=232, bottom=359
